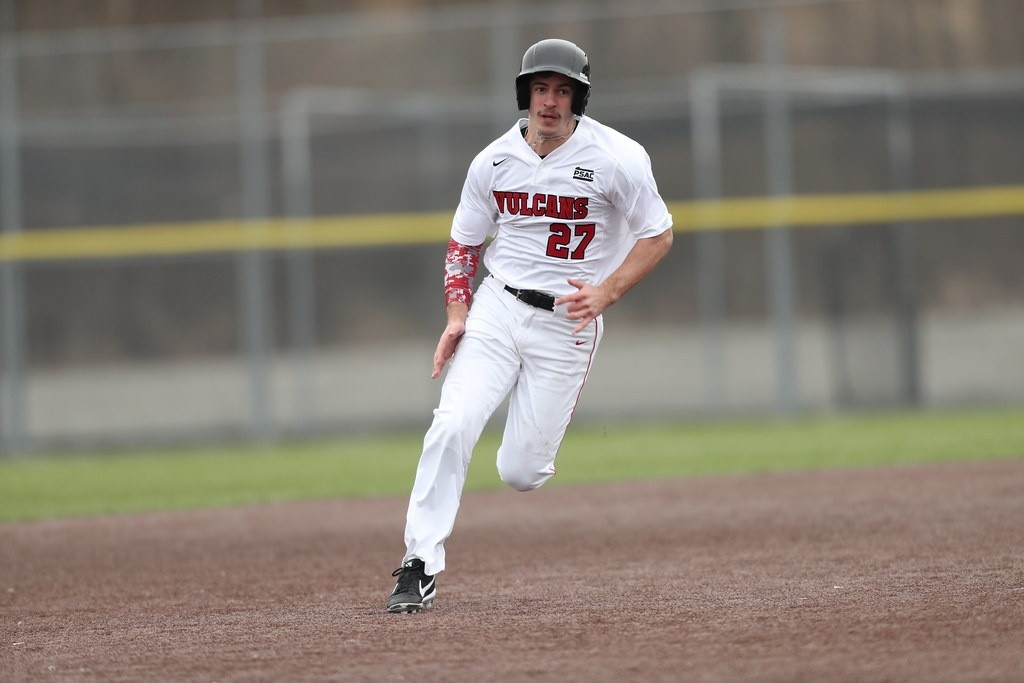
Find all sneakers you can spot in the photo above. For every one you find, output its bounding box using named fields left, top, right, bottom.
left=386, top=558, right=437, bottom=615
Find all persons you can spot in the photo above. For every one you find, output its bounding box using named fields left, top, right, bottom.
left=383, top=39, right=675, bottom=613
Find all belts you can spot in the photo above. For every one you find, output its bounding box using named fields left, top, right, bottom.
left=490, top=274, right=554, bottom=313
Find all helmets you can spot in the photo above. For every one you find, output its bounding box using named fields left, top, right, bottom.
left=514, top=39, right=592, bottom=116
